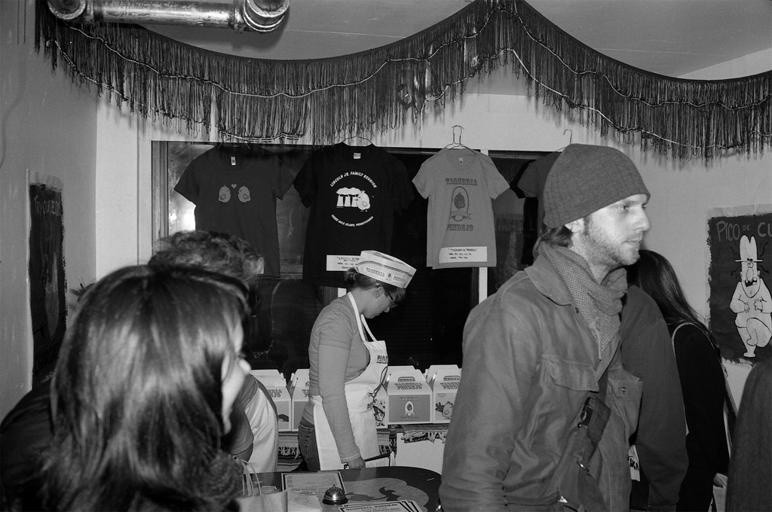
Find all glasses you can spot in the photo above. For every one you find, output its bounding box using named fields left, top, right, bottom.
left=383, top=288, right=398, bottom=309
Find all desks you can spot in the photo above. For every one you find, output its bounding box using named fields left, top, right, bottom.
left=239, top=466, right=442, bottom=512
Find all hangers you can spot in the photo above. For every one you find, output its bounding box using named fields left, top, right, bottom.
left=217, top=115, right=475, bottom=154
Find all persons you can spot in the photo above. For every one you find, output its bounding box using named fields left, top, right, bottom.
left=530, top=218, right=692, bottom=511
left=297, top=249, right=416, bottom=470
left=721, top=347, right=771, bottom=512
left=143, top=228, right=281, bottom=476
left=621, top=249, right=736, bottom=512
left=439, top=142, right=645, bottom=512
left=0, top=262, right=251, bottom=511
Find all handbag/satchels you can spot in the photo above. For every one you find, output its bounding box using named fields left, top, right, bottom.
left=233, top=460, right=288, bottom=511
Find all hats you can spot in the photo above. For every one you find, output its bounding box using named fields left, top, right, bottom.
left=356, top=249, right=418, bottom=290
left=542, top=143, right=652, bottom=229
